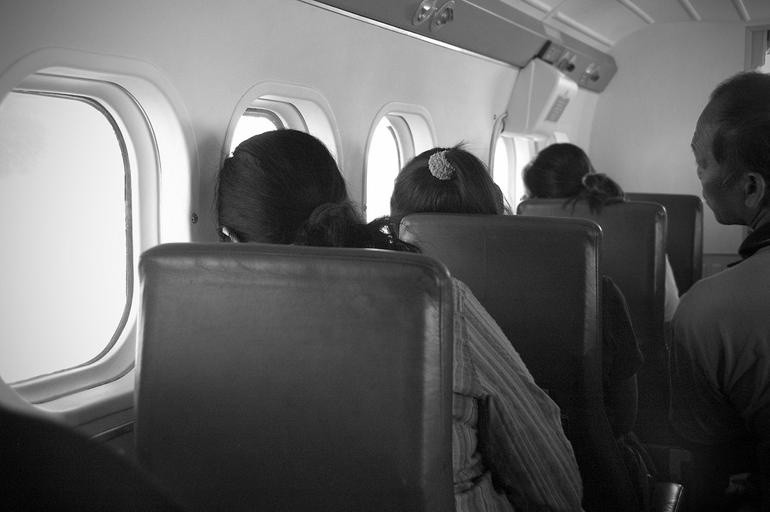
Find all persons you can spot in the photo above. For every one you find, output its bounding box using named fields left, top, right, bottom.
left=660, top=69, right=770, bottom=510
left=522, top=143, right=680, bottom=331
left=208, top=128, right=584, bottom=511
left=387, top=139, right=656, bottom=492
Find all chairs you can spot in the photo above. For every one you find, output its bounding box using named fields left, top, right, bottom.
left=395, top=209, right=608, bottom=504
left=124, top=239, right=458, bottom=508
left=622, top=191, right=702, bottom=294
left=511, top=195, right=673, bottom=512
